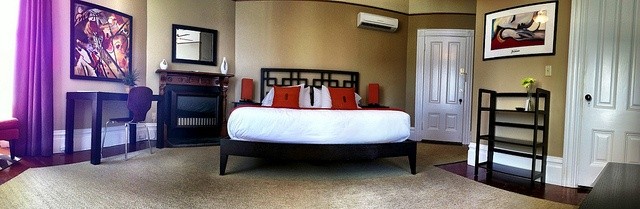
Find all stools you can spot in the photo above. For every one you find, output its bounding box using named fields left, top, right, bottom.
left=0, top=118, right=19, bottom=160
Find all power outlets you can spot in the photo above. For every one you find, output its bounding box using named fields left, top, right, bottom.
left=544, top=65, right=552, bottom=79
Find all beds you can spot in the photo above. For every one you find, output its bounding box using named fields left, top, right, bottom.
left=220, top=67, right=417, bottom=175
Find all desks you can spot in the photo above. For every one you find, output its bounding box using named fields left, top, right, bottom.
left=581, top=162, right=639, bottom=208
left=65, top=93, right=162, bottom=165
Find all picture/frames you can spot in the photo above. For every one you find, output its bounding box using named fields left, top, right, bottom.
left=483, top=1, right=557, bottom=60
left=69, top=0, right=132, bottom=82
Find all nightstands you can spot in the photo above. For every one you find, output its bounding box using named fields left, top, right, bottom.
left=361, top=105, right=390, bottom=108
left=231, top=101, right=261, bottom=107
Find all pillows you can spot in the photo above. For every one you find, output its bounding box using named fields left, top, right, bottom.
left=261, top=85, right=312, bottom=108
left=328, top=87, right=357, bottom=110
left=320, top=85, right=361, bottom=108
left=262, top=83, right=305, bottom=108
left=313, top=87, right=321, bottom=107
left=272, top=85, right=301, bottom=109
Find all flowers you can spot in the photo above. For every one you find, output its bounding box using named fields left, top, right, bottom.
left=522, top=76, right=535, bottom=93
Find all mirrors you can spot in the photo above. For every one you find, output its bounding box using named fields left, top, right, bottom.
left=171, top=23, right=218, bottom=65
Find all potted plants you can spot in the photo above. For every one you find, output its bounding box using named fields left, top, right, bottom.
left=124, top=71, right=139, bottom=89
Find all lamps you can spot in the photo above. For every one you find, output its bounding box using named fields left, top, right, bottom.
left=239, top=78, right=254, bottom=104
left=368, top=83, right=379, bottom=105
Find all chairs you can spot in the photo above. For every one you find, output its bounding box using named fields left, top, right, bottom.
left=100, top=86, right=153, bottom=160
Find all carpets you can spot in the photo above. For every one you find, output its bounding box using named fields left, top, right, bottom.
left=0, top=153, right=23, bottom=173
left=1, top=143, right=577, bottom=207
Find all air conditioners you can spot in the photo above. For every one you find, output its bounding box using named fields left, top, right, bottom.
left=357, top=12, right=399, bottom=33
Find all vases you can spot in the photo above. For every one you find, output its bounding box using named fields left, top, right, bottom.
left=525, top=93, right=533, bottom=109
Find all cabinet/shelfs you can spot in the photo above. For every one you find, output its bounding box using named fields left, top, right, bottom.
left=476, top=88, right=550, bottom=188
left=155, top=69, right=235, bottom=148
